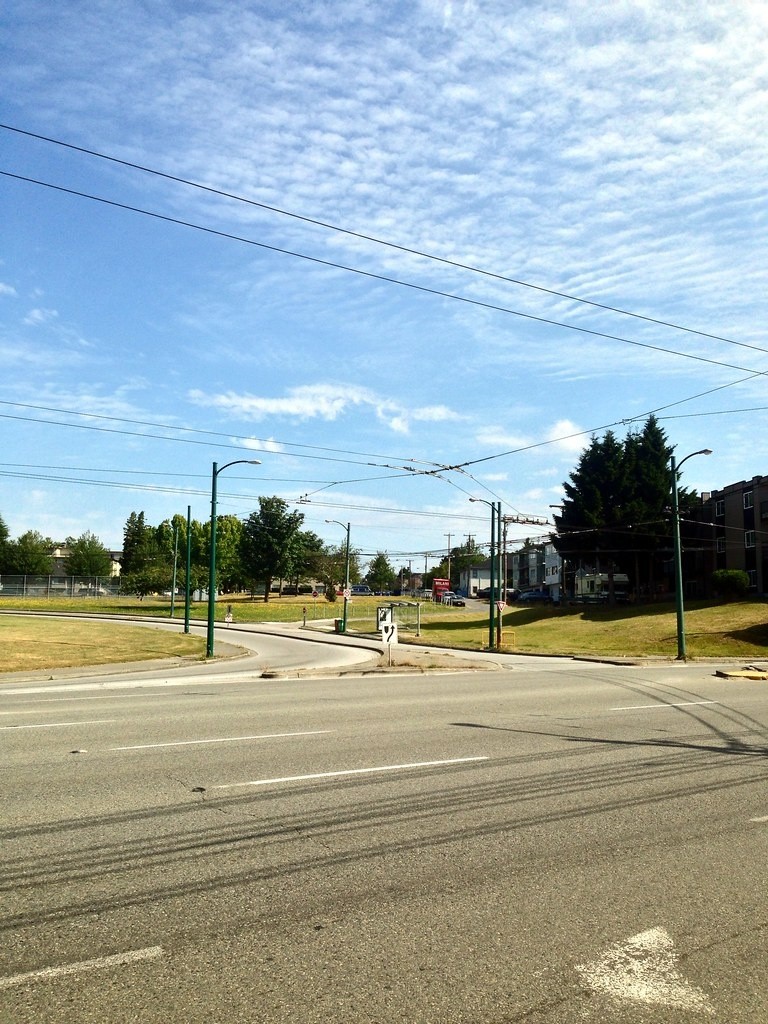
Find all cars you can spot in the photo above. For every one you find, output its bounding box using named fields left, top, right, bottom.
left=447, top=595, right=465, bottom=607
left=476, top=587, right=498, bottom=598
left=502, top=589, right=521, bottom=592
left=518, top=591, right=554, bottom=603
left=441, top=592, right=456, bottom=604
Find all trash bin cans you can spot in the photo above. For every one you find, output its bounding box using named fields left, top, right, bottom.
left=335, top=619, right=344, bottom=632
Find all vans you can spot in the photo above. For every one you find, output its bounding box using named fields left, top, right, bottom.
left=352, top=586, right=374, bottom=596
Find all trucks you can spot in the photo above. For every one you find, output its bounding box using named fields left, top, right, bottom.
left=432, top=579, right=450, bottom=602
left=574, top=573, right=629, bottom=604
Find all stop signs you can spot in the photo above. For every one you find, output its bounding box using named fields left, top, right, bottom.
left=312, top=591, right=318, bottom=598
left=302, top=607, right=306, bottom=614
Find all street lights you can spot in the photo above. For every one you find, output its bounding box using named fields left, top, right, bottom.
left=468, top=498, right=502, bottom=649
left=325, top=519, right=350, bottom=633
left=206, top=460, right=262, bottom=656
left=164, top=523, right=179, bottom=617
left=671, top=448, right=714, bottom=659
